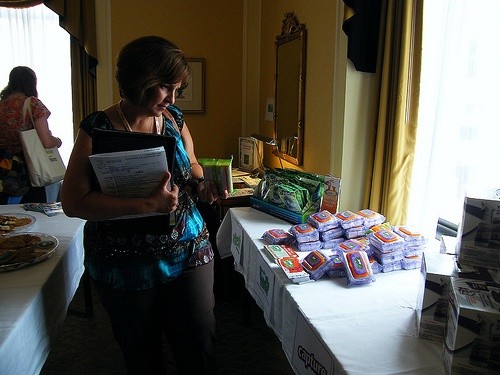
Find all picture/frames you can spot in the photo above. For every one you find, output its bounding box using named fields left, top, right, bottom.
left=176, top=58, right=207, bottom=114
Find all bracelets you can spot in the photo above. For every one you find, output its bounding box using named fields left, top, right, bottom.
left=198, top=178, right=208, bottom=184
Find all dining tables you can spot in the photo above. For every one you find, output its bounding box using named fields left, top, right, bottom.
left=0, top=204, right=87, bottom=375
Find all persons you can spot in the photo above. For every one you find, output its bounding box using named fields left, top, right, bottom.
left=60, top=36, right=219, bottom=375
left=0, top=67, right=62, bottom=204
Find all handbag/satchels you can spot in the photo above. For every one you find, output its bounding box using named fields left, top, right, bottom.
left=19, top=96, right=66, bottom=187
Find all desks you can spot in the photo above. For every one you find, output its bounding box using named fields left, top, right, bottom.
left=218, top=168, right=264, bottom=224
left=215, top=207, right=447, bottom=375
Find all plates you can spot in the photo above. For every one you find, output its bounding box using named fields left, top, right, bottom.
left=0, top=213, right=36, bottom=234
left=0, top=232, right=59, bottom=272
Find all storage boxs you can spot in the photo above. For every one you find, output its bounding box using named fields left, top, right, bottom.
left=251, top=190, right=500, bottom=375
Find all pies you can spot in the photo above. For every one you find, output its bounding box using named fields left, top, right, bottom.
left=0, top=215, right=17, bottom=223
left=0, top=233, right=41, bottom=266
left=13, top=217, right=31, bottom=226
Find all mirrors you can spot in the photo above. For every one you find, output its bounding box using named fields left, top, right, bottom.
left=273, top=12, right=307, bottom=167
